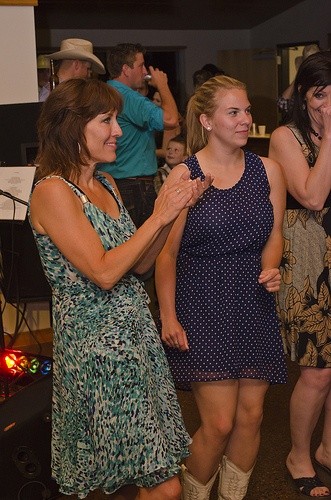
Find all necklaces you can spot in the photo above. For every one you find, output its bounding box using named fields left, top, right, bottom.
left=310, top=126, right=322, bottom=141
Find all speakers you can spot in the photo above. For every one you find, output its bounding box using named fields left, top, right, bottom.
left=0, top=102, right=44, bottom=167
left=0, top=375, right=63, bottom=500
left=0, top=219, right=52, bottom=304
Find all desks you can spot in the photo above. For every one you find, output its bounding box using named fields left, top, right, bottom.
left=248, top=130, right=272, bottom=158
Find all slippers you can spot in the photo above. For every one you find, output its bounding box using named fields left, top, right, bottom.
left=293, top=472, right=328, bottom=497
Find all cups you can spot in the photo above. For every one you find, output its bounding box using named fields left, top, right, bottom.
left=258, top=126, right=266, bottom=135
left=144, top=73, right=152, bottom=81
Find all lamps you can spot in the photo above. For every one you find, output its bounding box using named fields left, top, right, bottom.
left=0, top=347, right=53, bottom=380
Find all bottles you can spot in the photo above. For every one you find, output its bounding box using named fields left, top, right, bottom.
left=49, top=59, right=59, bottom=91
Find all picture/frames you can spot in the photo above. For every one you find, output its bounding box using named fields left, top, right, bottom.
left=91, top=43, right=184, bottom=117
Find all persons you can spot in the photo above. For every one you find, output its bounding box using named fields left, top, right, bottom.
left=27, top=78, right=215, bottom=500
left=37, top=39, right=331, bottom=500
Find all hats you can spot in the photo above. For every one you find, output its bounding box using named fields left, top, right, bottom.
left=44, top=38, right=106, bottom=74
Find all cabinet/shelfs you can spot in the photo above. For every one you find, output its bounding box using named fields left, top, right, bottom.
left=0, top=1, right=39, bottom=107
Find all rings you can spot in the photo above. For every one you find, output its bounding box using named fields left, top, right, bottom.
left=175, top=188, right=182, bottom=194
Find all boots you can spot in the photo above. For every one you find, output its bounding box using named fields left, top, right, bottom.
left=218, top=455, right=256, bottom=500
left=180, top=463, right=222, bottom=500
